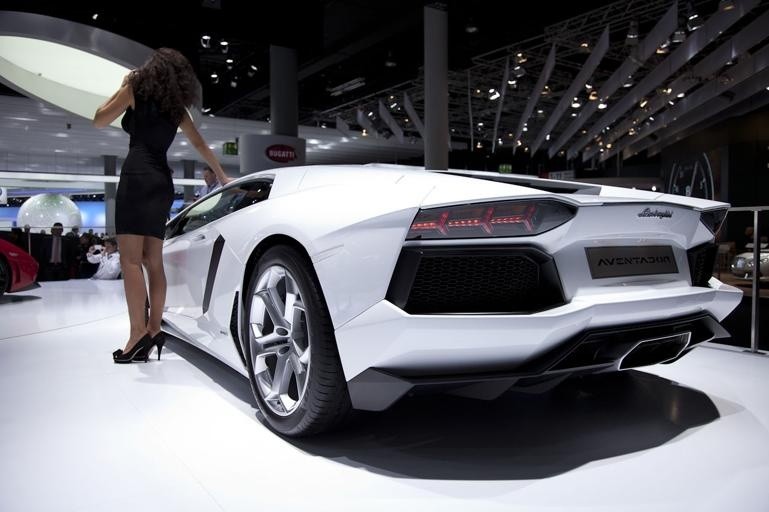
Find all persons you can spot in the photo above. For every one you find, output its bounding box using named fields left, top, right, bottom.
left=735, top=227, right=753, bottom=254
left=191, top=164, right=219, bottom=201
left=93, top=47, right=235, bottom=366
left=183, top=185, right=269, bottom=235
left=40, top=222, right=124, bottom=281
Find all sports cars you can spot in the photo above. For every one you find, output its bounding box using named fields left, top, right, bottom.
left=141, top=153, right=752, bottom=448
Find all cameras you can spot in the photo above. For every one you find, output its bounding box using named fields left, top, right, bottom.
left=94, top=245, right=102, bottom=250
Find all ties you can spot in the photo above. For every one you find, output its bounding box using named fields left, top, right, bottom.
left=54, top=236, right=60, bottom=265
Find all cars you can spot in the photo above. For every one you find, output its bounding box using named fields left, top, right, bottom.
left=730, top=249, right=769, bottom=280
left=0, top=236, right=41, bottom=298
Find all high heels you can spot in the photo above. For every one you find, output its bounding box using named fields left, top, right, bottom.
left=112, top=332, right=152, bottom=364
left=133, top=330, right=168, bottom=361
